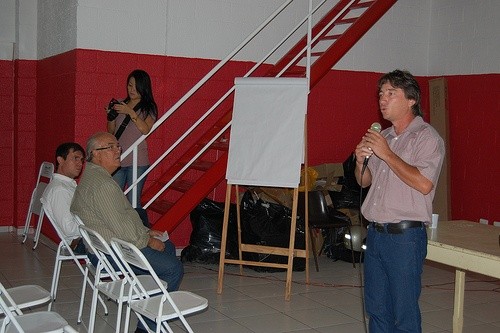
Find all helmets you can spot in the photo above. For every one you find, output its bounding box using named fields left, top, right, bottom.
left=342, top=225, right=369, bottom=252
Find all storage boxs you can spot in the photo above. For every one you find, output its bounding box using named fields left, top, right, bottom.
left=314, top=164, right=344, bottom=177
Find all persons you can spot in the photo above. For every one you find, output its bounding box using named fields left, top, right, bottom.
left=354, top=70, right=446, bottom=333
left=104, top=69, right=159, bottom=210
left=69, top=132, right=184, bottom=333
left=41, top=142, right=86, bottom=257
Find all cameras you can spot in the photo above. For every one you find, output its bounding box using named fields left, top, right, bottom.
left=107, top=97, right=120, bottom=121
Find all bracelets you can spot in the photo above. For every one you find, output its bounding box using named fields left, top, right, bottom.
left=131, top=116, right=139, bottom=123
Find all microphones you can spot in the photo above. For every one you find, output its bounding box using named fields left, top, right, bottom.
left=363, top=122, right=382, bottom=166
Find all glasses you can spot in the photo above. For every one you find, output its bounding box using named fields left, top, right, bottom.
left=95, top=145, right=121, bottom=151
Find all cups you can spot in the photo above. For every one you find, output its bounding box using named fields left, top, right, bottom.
left=428, top=213, right=439, bottom=228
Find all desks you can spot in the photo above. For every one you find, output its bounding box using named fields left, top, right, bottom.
left=426, top=220, right=500, bottom=333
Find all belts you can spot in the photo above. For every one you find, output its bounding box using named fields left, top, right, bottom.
left=370, top=220, right=424, bottom=235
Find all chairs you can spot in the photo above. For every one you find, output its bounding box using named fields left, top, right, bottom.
left=0, top=161, right=208, bottom=333
left=297, top=189, right=356, bottom=272
